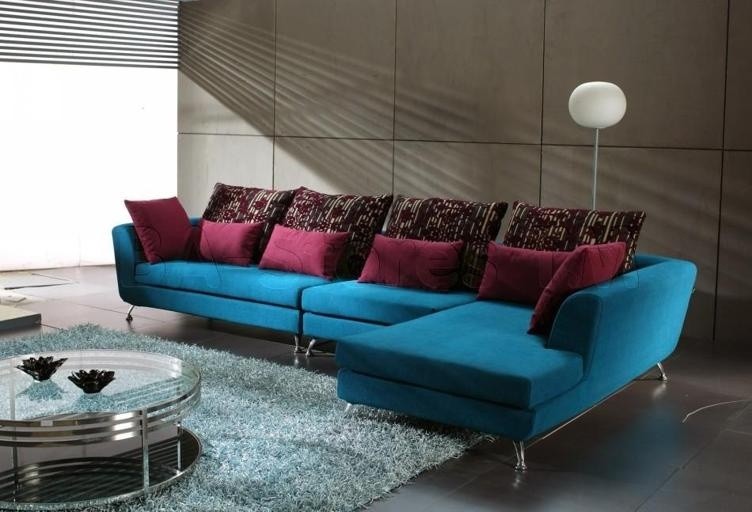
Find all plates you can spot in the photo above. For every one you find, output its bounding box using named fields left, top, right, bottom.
left=15, top=356, right=69, bottom=381
left=68, top=370, right=115, bottom=394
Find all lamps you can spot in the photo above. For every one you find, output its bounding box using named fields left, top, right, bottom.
left=568, top=81, right=628, bottom=210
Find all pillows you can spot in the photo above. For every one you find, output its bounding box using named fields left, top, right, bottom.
left=381, top=194, right=507, bottom=296
left=357, top=232, right=465, bottom=292
left=477, top=240, right=572, bottom=309
left=189, top=220, right=264, bottom=265
left=524, top=243, right=626, bottom=335
left=502, top=201, right=645, bottom=278
left=124, top=197, right=194, bottom=265
left=259, top=224, right=351, bottom=277
left=261, top=185, right=392, bottom=282
left=200, top=182, right=296, bottom=265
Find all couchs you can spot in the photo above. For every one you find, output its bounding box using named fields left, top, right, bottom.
left=111, top=181, right=699, bottom=470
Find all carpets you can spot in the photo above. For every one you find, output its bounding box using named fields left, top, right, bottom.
left=0, top=324, right=505, bottom=512
